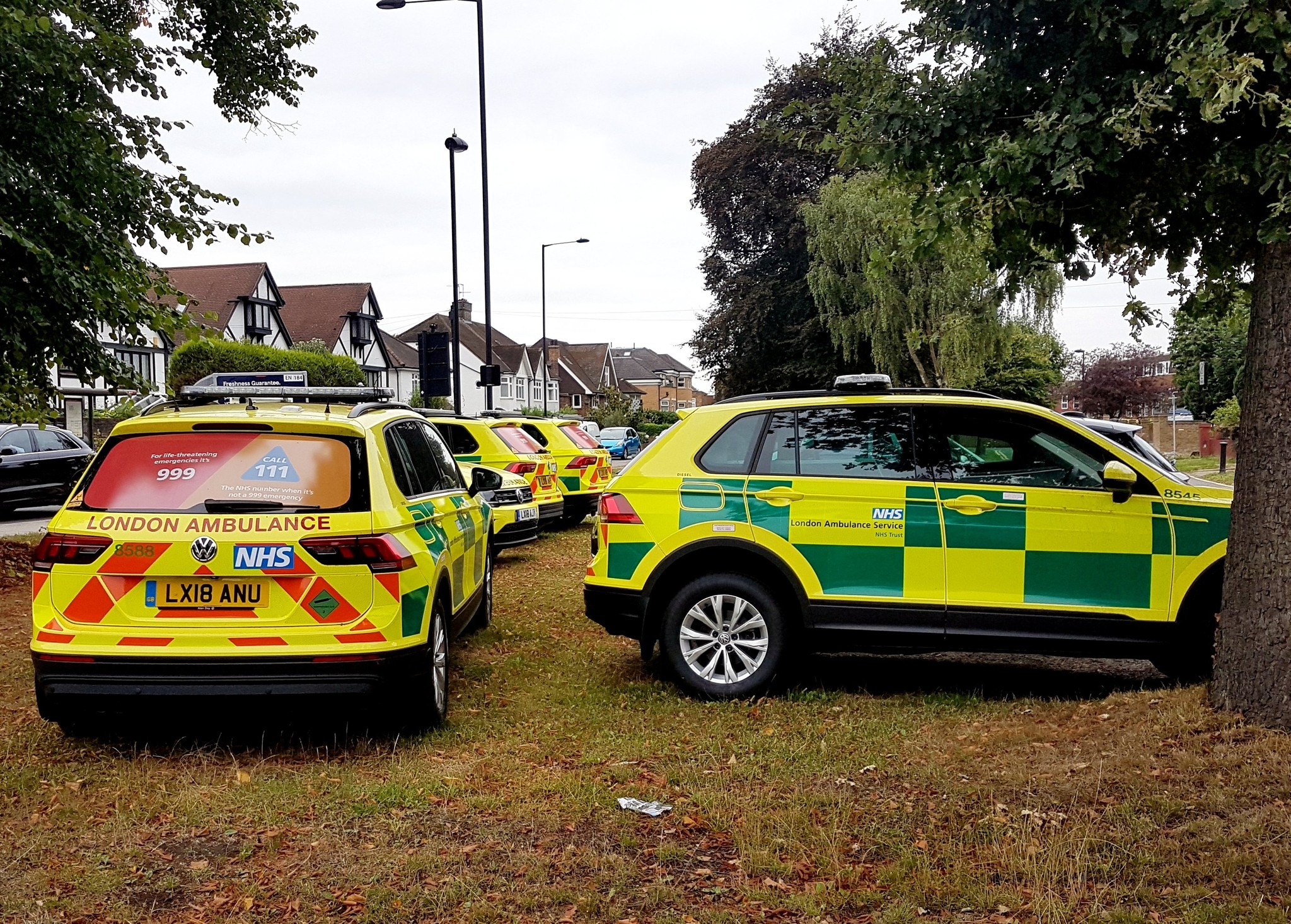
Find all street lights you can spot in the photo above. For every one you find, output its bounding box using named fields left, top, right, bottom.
left=445, top=129, right=468, bottom=417
left=1075, top=350, right=1084, bottom=411
left=542, top=239, right=589, bottom=416
left=376, top=0, right=494, bottom=411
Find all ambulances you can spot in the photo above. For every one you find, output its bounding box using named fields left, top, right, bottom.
left=412, top=405, right=610, bottom=553
left=581, top=387, right=1232, bottom=704
left=30, top=400, right=504, bottom=731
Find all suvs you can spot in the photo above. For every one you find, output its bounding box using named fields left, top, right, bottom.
left=0, top=423, right=97, bottom=521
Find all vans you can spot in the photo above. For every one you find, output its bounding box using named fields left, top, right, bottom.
left=554, top=417, right=601, bottom=444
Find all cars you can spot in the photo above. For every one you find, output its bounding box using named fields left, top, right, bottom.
left=600, top=427, right=641, bottom=460
